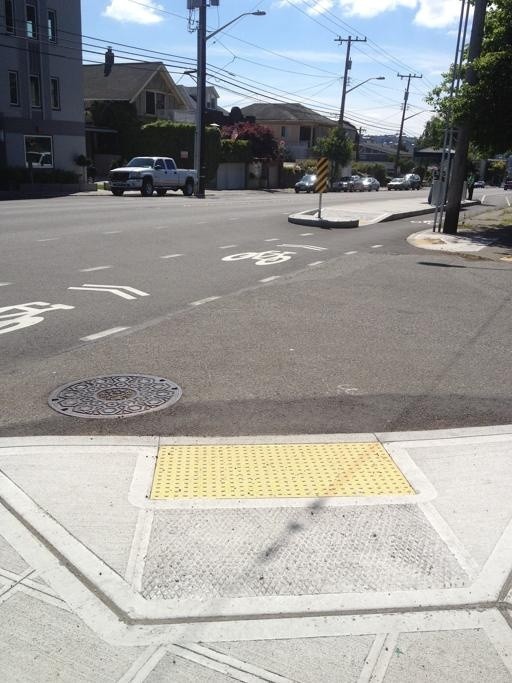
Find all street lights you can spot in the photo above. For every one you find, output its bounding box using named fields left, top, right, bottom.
left=194, top=11, right=266, bottom=200
left=394, top=110, right=436, bottom=178
left=334, top=77, right=385, bottom=181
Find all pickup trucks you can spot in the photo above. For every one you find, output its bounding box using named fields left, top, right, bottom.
left=109, top=156, right=197, bottom=197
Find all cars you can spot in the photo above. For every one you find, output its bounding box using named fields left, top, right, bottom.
left=332, top=174, right=380, bottom=192
left=387, top=173, right=422, bottom=191
left=473, top=180, right=485, bottom=188
left=294, top=174, right=331, bottom=193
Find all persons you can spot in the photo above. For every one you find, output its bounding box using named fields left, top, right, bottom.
left=466, top=171, right=475, bottom=200
